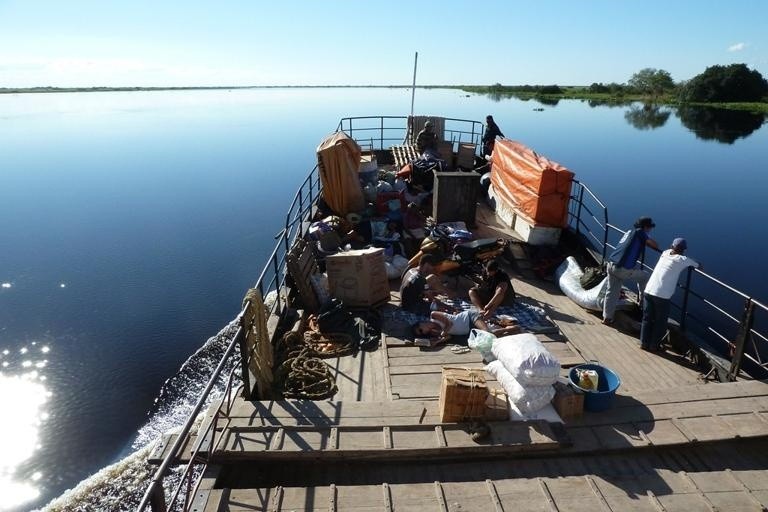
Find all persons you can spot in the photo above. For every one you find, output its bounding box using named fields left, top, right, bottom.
left=482, top=115, right=505, bottom=162
left=413, top=308, right=521, bottom=349
left=640, top=238, right=702, bottom=350
left=415, top=121, right=439, bottom=152
left=469, top=259, right=514, bottom=318
left=400, top=253, right=449, bottom=316
left=602, top=216, right=658, bottom=325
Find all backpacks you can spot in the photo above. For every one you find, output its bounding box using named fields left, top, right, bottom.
left=580, top=263, right=607, bottom=289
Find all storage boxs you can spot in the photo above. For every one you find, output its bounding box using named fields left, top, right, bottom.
left=551, top=382, right=585, bottom=417
left=439, top=365, right=490, bottom=424
left=325, top=248, right=391, bottom=312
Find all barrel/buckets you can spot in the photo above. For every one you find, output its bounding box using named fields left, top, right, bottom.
left=570, top=364, right=620, bottom=411
left=359, top=161, right=379, bottom=201
left=392, top=253, right=410, bottom=272
left=574, top=368, right=599, bottom=390
left=577, top=372, right=593, bottom=390
left=383, top=260, right=400, bottom=280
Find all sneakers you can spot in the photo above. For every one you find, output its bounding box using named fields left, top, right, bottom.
left=602, top=316, right=618, bottom=324
left=641, top=345, right=662, bottom=351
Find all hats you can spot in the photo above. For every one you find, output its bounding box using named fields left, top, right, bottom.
left=671, top=237, right=688, bottom=250
left=634, top=215, right=655, bottom=229
left=425, top=121, right=435, bottom=127
